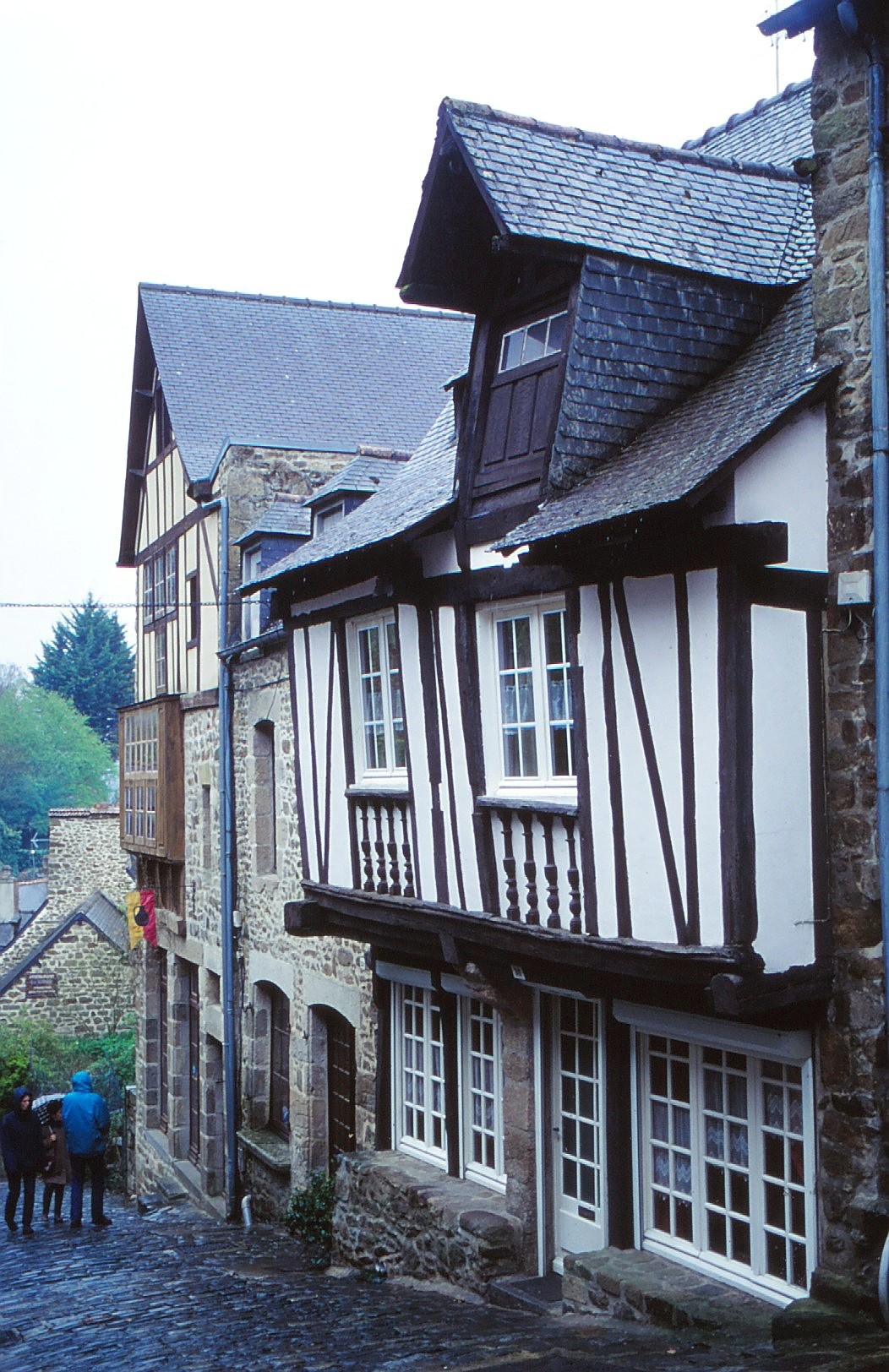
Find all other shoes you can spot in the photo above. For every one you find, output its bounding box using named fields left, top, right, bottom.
left=54, top=1216, right=63, bottom=1223
left=22, top=1228, right=34, bottom=1236
left=70, top=1221, right=82, bottom=1228
left=92, top=1216, right=112, bottom=1225
left=4, top=1217, right=18, bottom=1231
left=40, top=1215, right=48, bottom=1221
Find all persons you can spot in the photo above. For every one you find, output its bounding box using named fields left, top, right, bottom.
left=41, top=1101, right=69, bottom=1222
left=62, top=1070, right=111, bottom=1228
left=0, top=1087, right=45, bottom=1235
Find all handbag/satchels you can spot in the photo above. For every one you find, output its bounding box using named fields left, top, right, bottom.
left=41, top=1158, right=61, bottom=1177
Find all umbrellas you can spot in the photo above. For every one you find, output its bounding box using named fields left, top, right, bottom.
left=32, top=1093, right=65, bottom=1136
